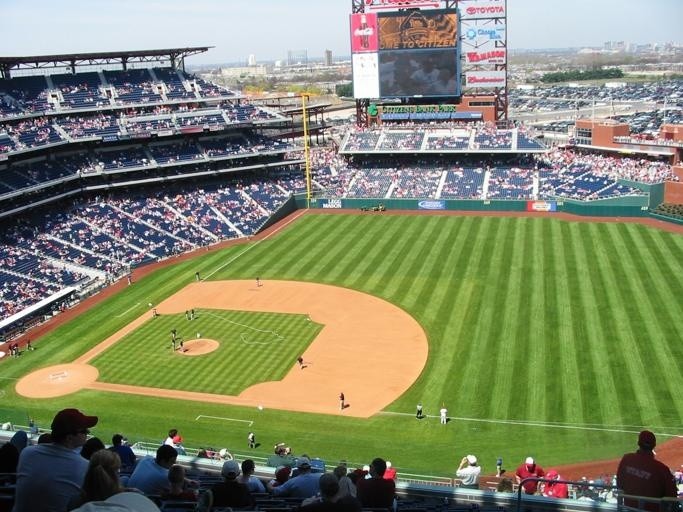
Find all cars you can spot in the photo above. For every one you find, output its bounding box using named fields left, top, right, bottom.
left=507, top=78, right=682, bottom=139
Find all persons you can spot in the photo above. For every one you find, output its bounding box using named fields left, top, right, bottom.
left=309, top=120, right=682, bottom=212
left=416, top=403, right=422, bottom=418
left=297, top=355, right=303, bottom=366
left=339, top=392, right=346, bottom=410
left=8, top=338, right=32, bottom=356
left=170, top=328, right=184, bottom=352
left=0, top=71, right=321, bottom=343
left=1, top=408, right=682, bottom=512
left=378, top=50, right=457, bottom=97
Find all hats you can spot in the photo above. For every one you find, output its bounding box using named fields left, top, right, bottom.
left=350, top=469, right=368, bottom=484
left=465, top=454, right=478, bottom=464
left=295, top=456, right=312, bottom=468
left=639, top=429, right=656, bottom=447
left=525, top=456, right=536, bottom=468
left=221, top=459, right=240, bottom=480
left=50, top=407, right=98, bottom=431
left=543, top=469, right=559, bottom=482
left=274, top=466, right=291, bottom=477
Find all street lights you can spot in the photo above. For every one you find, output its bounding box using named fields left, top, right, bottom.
left=300, top=92, right=320, bottom=208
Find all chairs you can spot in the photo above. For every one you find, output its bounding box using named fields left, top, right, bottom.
left=287, top=125, right=634, bottom=214
left=0, top=45, right=292, bottom=346
left=0, top=404, right=680, bottom=512
